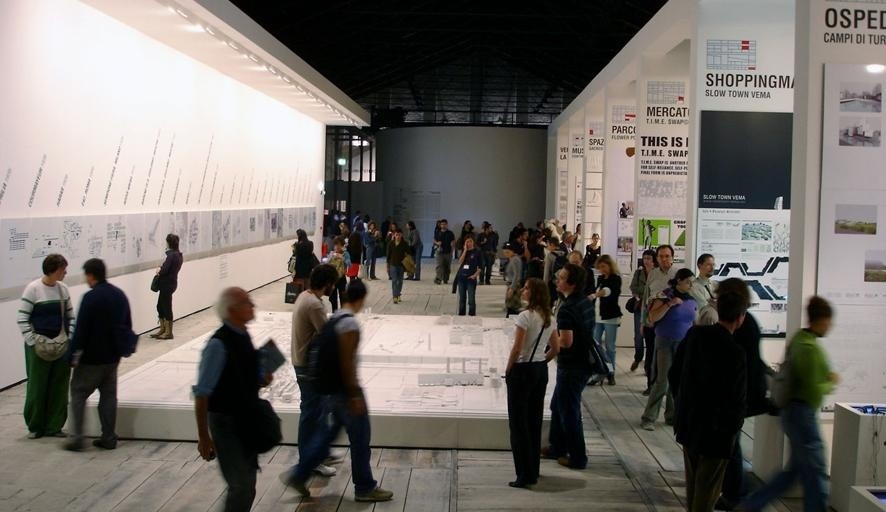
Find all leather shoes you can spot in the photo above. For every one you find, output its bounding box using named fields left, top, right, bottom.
left=509, top=474, right=537, bottom=487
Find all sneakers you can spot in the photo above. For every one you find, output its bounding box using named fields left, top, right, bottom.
left=631, top=360, right=655, bottom=430
left=313, top=463, right=337, bottom=476
left=394, top=298, right=398, bottom=304
left=398, top=296, right=401, bottom=301
left=541, top=445, right=563, bottom=460
left=355, top=483, right=392, bottom=501
left=557, top=455, right=587, bottom=469
left=278, top=473, right=310, bottom=496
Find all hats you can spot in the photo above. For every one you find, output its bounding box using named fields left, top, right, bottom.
left=502, top=242, right=514, bottom=250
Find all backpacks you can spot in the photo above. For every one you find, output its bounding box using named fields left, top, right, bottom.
left=773, top=358, right=800, bottom=407
left=297, top=313, right=352, bottom=395
left=551, top=250, right=568, bottom=275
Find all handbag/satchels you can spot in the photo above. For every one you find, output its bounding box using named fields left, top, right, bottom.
left=150, top=275, right=160, bottom=292
left=511, top=364, right=526, bottom=384
left=285, top=283, right=302, bottom=303
left=32, top=332, right=68, bottom=361
left=246, top=399, right=281, bottom=454
left=625, top=296, right=641, bottom=313
left=585, top=338, right=614, bottom=384
left=402, top=256, right=416, bottom=275
left=358, top=265, right=365, bottom=278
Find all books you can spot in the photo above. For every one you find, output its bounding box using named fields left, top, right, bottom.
left=257, top=339, right=286, bottom=375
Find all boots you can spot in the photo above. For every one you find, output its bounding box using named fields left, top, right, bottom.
left=157, top=320, right=173, bottom=338
left=150, top=317, right=164, bottom=337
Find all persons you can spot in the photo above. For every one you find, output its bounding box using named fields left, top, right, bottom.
left=540, top=263, right=596, bottom=469
left=16, top=253, right=76, bottom=438
left=149, top=236, right=183, bottom=340
left=430, top=219, right=498, bottom=315
left=291, top=264, right=344, bottom=476
left=328, top=209, right=423, bottom=305
left=58, top=258, right=138, bottom=452
left=292, top=229, right=320, bottom=278
left=192, top=287, right=272, bottom=512
left=279, top=279, right=393, bottom=501
left=625, top=244, right=839, bottom=511
left=505, top=277, right=560, bottom=488
left=501, top=220, right=601, bottom=318
left=587, top=255, right=623, bottom=386
